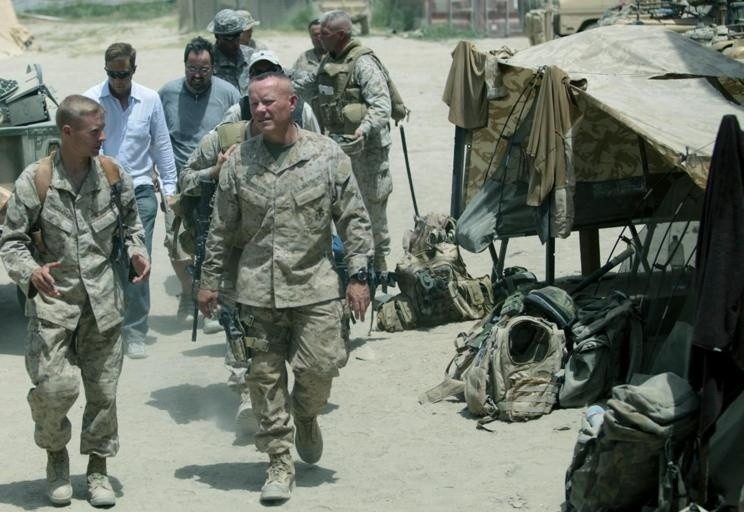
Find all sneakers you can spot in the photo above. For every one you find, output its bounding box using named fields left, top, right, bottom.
left=123, top=338, right=152, bottom=360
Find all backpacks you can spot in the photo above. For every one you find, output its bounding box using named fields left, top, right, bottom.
left=398, top=248, right=475, bottom=328
left=461, top=311, right=569, bottom=423
left=409, top=214, right=470, bottom=279
left=564, top=372, right=705, bottom=512
left=556, top=290, right=644, bottom=410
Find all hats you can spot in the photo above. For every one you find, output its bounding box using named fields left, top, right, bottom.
left=206, top=8, right=245, bottom=34
left=235, top=9, right=262, bottom=31
left=243, top=48, right=284, bottom=74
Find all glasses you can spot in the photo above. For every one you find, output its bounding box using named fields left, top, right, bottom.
left=185, top=64, right=213, bottom=74
left=104, top=66, right=134, bottom=79
left=219, top=34, right=242, bottom=41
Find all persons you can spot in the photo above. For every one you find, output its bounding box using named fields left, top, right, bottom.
left=0, top=95, right=150, bottom=507
left=181, top=50, right=322, bottom=421
left=82, top=42, right=178, bottom=358
left=196, top=70, right=376, bottom=503
left=281, top=10, right=393, bottom=280
left=156, top=9, right=272, bottom=335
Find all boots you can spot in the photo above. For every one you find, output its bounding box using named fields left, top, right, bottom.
left=176, top=291, right=201, bottom=328
left=43, top=446, right=75, bottom=506
left=83, top=456, right=117, bottom=507
left=203, top=310, right=227, bottom=334
left=232, top=385, right=262, bottom=439
left=292, top=411, right=325, bottom=464
left=259, top=450, right=297, bottom=502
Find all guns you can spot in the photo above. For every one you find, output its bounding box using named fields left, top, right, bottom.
left=190, top=179, right=218, bottom=342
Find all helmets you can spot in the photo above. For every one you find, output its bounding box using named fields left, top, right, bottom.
left=525, top=282, right=578, bottom=332
